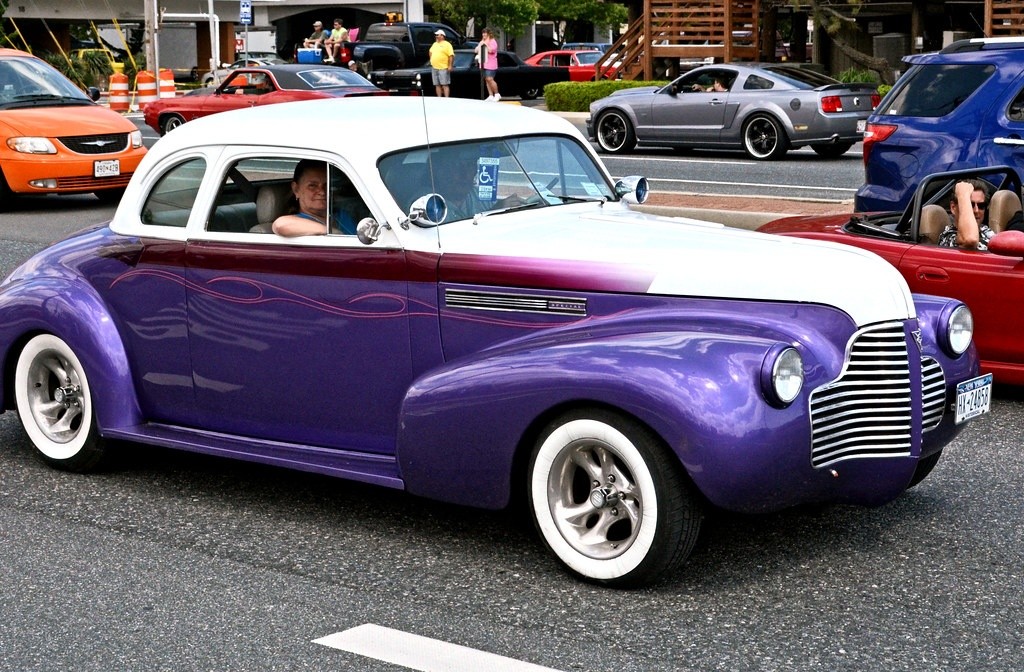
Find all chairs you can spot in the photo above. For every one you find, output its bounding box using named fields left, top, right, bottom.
left=920, top=205, right=949, bottom=246
left=349, top=28, right=361, bottom=43
left=385, top=161, right=427, bottom=214
left=989, top=189, right=1021, bottom=237
left=250, top=183, right=296, bottom=233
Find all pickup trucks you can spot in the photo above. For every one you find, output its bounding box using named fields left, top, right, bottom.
left=293, top=23, right=479, bottom=72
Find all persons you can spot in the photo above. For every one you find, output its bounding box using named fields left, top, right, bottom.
left=304, top=21, right=329, bottom=55
left=271, top=159, right=357, bottom=236
left=475, top=27, right=502, bottom=101
left=407, top=147, right=527, bottom=225
left=234, top=74, right=274, bottom=95
left=429, top=30, right=455, bottom=97
left=939, top=180, right=996, bottom=252
left=692, top=75, right=728, bottom=92
left=324, top=19, right=351, bottom=63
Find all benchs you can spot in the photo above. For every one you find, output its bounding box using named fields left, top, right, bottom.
left=152, top=201, right=256, bottom=232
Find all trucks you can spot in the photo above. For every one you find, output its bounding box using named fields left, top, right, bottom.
left=77, top=25, right=277, bottom=81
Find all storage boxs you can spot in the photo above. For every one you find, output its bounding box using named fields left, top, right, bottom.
left=297, top=48, right=322, bottom=65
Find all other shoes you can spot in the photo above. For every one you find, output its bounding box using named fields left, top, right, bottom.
left=485, top=93, right=502, bottom=102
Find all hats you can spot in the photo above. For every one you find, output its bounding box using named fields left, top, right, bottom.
left=435, top=30, right=445, bottom=36
left=313, top=22, right=321, bottom=26
left=349, top=61, right=355, bottom=66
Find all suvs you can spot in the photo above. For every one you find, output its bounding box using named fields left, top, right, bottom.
left=853, top=36, right=1024, bottom=213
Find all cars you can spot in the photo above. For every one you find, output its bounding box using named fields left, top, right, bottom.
left=0, top=96, right=994, bottom=592
left=524, top=43, right=627, bottom=82
left=69, top=49, right=124, bottom=74
left=200, top=51, right=290, bottom=89
left=70, top=33, right=96, bottom=50
left=0, top=48, right=149, bottom=212
left=367, top=49, right=570, bottom=100
left=143, top=64, right=421, bottom=137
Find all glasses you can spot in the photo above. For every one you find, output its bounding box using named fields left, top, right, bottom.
left=971, top=202, right=986, bottom=210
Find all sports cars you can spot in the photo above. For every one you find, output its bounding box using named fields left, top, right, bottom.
left=585, top=62, right=882, bottom=161
left=755, top=164, right=1024, bottom=388
